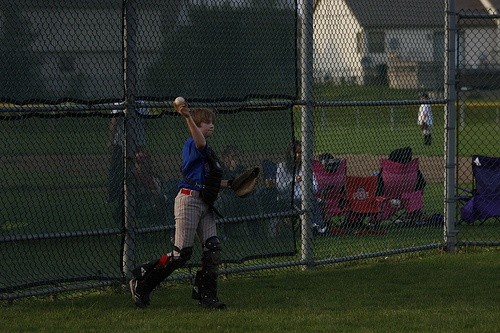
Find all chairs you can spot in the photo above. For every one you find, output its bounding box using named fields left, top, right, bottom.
left=454, top=156, right=500, bottom=226
left=312, top=150, right=424, bottom=231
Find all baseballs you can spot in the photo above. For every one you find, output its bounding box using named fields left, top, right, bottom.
left=175, top=97, right=185, bottom=105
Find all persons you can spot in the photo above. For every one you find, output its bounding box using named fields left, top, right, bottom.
left=376, top=147, right=425, bottom=196
left=130, top=103, right=260, bottom=311
left=275, top=140, right=330, bottom=236
left=416, top=92, right=432, bottom=146
left=107, top=110, right=159, bottom=226
left=218, top=146, right=261, bottom=241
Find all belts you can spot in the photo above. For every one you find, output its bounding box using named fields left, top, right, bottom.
left=181, top=189, right=191, bottom=196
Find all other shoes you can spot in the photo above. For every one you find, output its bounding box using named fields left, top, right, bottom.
left=312, top=222, right=327, bottom=234
left=129, top=279, right=149, bottom=309
left=199, top=298, right=226, bottom=310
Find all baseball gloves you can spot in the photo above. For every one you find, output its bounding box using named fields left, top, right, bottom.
left=233, top=166, right=262, bottom=197
